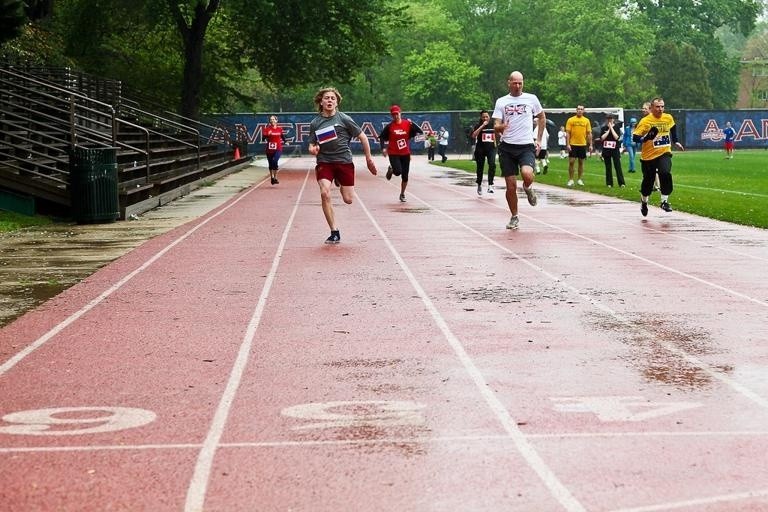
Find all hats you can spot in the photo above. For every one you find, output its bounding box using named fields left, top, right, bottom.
left=606, top=114, right=615, bottom=119
left=389, top=104, right=401, bottom=115
left=630, top=118, right=639, bottom=123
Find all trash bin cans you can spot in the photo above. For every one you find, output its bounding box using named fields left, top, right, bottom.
left=63, top=144, right=121, bottom=225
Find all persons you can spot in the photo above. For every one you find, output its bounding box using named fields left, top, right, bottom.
left=438, top=127, right=448, bottom=162
left=381, top=105, right=423, bottom=202
left=632, top=98, right=684, bottom=217
left=308, top=88, right=378, bottom=244
left=491, top=71, right=546, bottom=230
left=565, top=105, right=593, bottom=185
left=470, top=111, right=497, bottom=196
left=262, top=115, right=288, bottom=184
left=623, top=118, right=641, bottom=173
left=723, top=121, right=736, bottom=158
left=532, top=116, right=569, bottom=174
left=633, top=102, right=660, bottom=192
left=428, top=134, right=437, bottom=161
left=600, top=115, right=626, bottom=188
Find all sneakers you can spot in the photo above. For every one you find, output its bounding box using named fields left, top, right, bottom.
left=428, top=155, right=448, bottom=162
left=659, top=201, right=672, bottom=213
left=399, top=194, right=407, bottom=203
left=476, top=185, right=494, bottom=198
left=627, top=169, right=635, bottom=173
left=535, top=153, right=569, bottom=174
left=522, top=183, right=537, bottom=207
left=270, top=177, right=279, bottom=184
left=567, top=181, right=574, bottom=186
left=385, top=166, right=393, bottom=181
left=324, top=230, right=341, bottom=245
left=578, top=180, right=584, bottom=186
left=639, top=199, right=649, bottom=218
left=505, top=214, right=520, bottom=230
left=605, top=184, right=626, bottom=190
